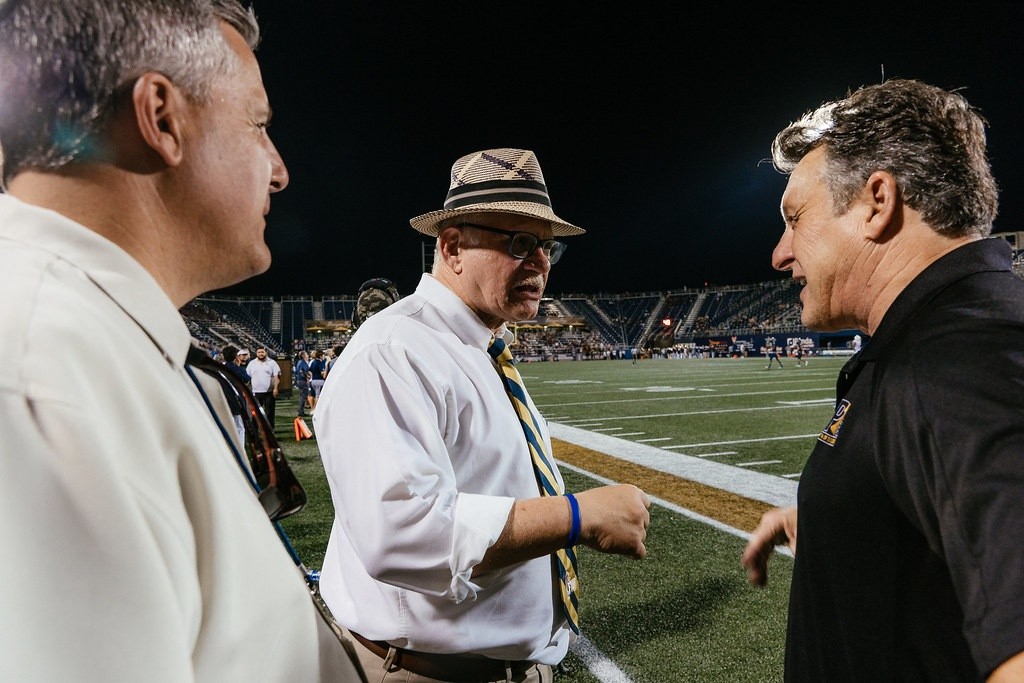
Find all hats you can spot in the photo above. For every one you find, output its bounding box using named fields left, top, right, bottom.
left=237, top=350, right=248, bottom=356
left=410, top=148, right=587, bottom=236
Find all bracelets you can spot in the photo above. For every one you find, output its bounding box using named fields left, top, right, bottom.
left=562, top=494, right=580, bottom=544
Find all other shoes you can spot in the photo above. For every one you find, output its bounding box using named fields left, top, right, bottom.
left=795, top=365, right=801, bottom=368
left=805, top=361, right=808, bottom=366
left=298, top=413, right=310, bottom=417
left=765, top=366, right=769, bottom=370
left=778, top=366, right=784, bottom=369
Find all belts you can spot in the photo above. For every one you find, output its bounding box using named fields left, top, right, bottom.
left=347, top=628, right=536, bottom=683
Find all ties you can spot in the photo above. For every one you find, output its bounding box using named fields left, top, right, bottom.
left=488, top=338, right=579, bottom=635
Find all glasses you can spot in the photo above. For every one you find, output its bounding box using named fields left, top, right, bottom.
left=457, top=221, right=569, bottom=265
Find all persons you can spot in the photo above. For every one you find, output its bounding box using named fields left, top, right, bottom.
left=0, top=0, right=368, bottom=683
left=744, top=80, right=1024, bottom=683
left=185, top=280, right=401, bottom=440
left=503, top=282, right=860, bottom=370
left=313, top=146, right=649, bottom=683
